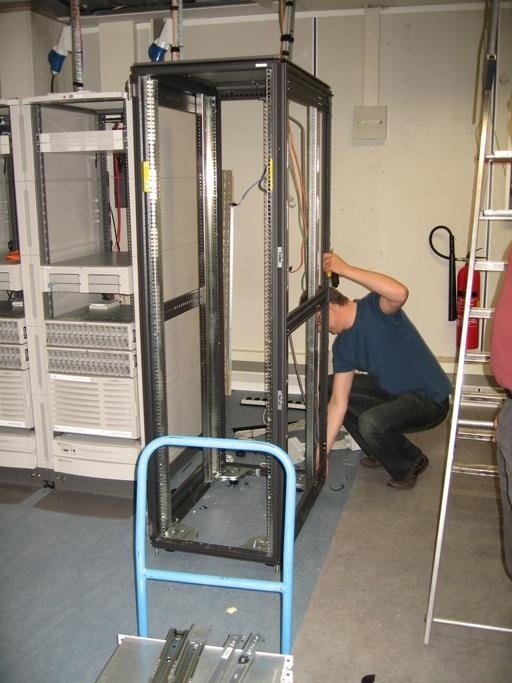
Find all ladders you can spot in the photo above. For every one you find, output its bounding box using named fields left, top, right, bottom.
left=424, top=91, right=512, bottom=645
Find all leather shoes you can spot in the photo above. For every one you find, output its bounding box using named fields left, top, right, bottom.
left=386, top=452, right=430, bottom=491
left=359, top=456, right=382, bottom=468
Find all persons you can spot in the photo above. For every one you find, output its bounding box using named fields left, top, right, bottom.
left=489, top=240, right=512, bottom=583
left=299, top=253, right=452, bottom=490
